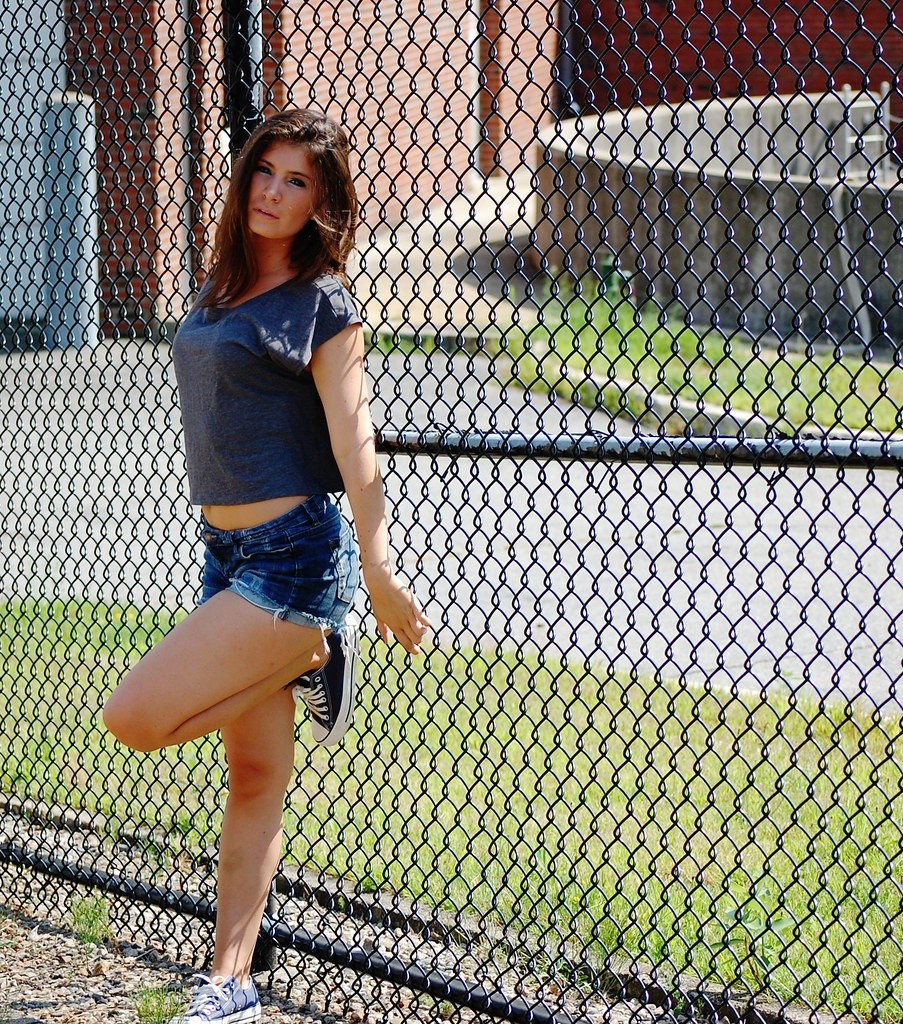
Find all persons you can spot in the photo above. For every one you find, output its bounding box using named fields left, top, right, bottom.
left=102, top=108, right=429, bottom=1024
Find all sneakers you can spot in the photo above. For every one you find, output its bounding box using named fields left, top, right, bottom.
left=166, top=973, right=262, bottom=1024
left=291, top=613, right=361, bottom=748
